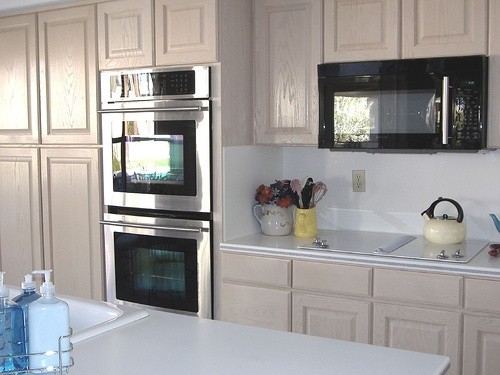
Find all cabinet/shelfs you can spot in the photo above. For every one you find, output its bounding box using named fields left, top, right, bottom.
left=324, top=0, right=489, bottom=62
left=252, top=0, right=320, bottom=144
left=461, top=265, right=500, bottom=374
left=218, top=243, right=291, bottom=330
left=1, top=148, right=103, bottom=302
left=0, top=5, right=98, bottom=146
left=373, top=253, right=463, bottom=375
left=293, top=250, right=373, bottom=344
left=96, top=0, right=220, bottom=72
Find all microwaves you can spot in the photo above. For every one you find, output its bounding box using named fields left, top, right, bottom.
left=318, top=55, right=486, bottom=152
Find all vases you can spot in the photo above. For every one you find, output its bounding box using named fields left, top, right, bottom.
left=249, top=203, right=293, bottom=235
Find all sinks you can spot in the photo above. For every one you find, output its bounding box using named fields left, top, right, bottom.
left=0, top=287, right=121, bottom=336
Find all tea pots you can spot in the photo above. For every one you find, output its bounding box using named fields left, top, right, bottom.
left=490, top=213, right=499, bottom=232
left=421, top=199, right=465, bottom=243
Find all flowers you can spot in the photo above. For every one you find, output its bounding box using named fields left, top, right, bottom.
left=252, top=180, right=295, bottom=206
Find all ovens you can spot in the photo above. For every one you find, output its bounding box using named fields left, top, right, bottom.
left=100, top=66, right=212, bottom=320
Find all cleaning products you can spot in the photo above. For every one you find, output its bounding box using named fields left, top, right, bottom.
left=27, top=269, right=71, bottom=375
left=12, top=274, right=42, bottom=371
left=0, top=271, right=26, bottom=375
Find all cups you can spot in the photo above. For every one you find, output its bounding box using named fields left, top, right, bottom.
left=295, top=208, right=317, bottom=238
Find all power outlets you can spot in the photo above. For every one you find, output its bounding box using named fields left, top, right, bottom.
left=351, top=169, right=366, bottom=193
